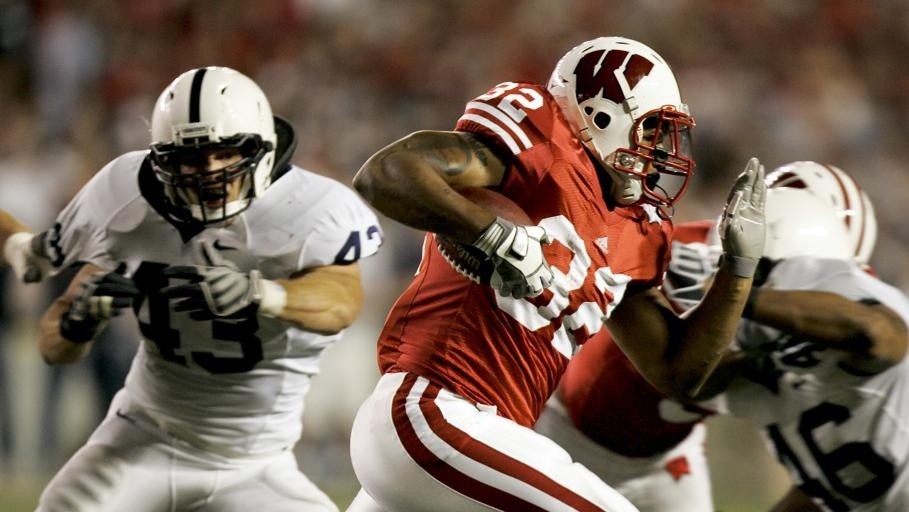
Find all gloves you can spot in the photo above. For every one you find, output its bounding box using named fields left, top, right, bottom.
left=156, top=236, right=266, bottom=330
left=468, top=215, right=558, bottom=302
left=719, top=156, right=772, bottom=279
left=1, top=219, right=66, bottom=289
left=60, top=255, right=149, bottom=345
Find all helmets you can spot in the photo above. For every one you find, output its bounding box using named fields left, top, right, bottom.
left=545, top=31, right=692, bottom=206
left=762, top=159, right=881, bottom=271
left=145, top=63, right=278, bottom=229
left=705, top=183, right=858, bottom=261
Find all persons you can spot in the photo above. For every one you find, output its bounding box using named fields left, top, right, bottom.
left=342, top=39, right=768, bottom=512
left=531, top=160, right=879, bottom=512
left=659, top=187, right=909, bottom=511
left=34, top=65, right=383, bottom=512
left=0, top=208, right=58, bottom=286
